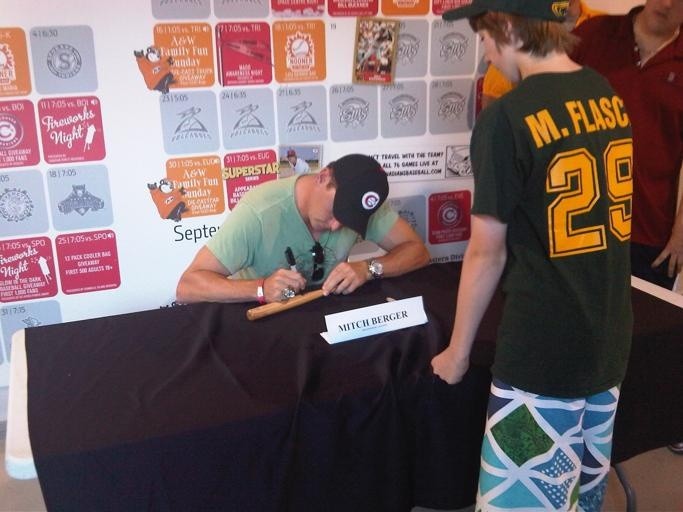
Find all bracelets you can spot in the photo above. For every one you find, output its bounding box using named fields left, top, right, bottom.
left=257, top=278, right=265, bottom=303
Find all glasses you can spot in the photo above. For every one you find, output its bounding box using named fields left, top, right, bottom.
left=309, top=242, right=326, bottom=282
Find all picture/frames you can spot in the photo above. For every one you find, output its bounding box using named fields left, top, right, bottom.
left=351, top=17, right=401, bottom=85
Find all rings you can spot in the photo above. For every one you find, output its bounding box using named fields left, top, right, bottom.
left=281, top=286, right=295, bottom=301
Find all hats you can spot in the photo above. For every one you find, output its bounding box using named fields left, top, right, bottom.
left=331, top=155, right=390, bottom=239
left=441, top=0, right=569, bottom=23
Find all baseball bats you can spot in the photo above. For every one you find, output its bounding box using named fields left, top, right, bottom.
left=247, top=285, right=339, bottom=321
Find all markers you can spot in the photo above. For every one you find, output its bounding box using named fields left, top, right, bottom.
left=285, top=247, right=306, bottom=296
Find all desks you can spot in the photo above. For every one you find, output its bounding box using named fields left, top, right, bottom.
left=7, top=253, right=682, bottom=512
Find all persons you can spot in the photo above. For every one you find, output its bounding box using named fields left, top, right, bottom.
left=176, top=154, right=432, bottom=303
left=481, top=0, right=608, bottom=110
left=287, top=150, right=311, bottom=176
left=567, top=0, right=683, bottom=453
left=430, top=0, right=635, bottom=512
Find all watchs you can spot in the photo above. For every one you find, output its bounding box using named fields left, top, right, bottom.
left=367, top=257, right=384, bottom=280
